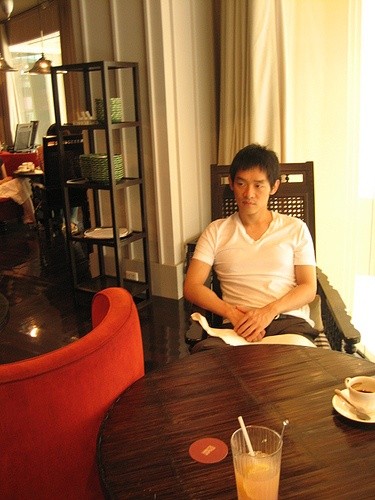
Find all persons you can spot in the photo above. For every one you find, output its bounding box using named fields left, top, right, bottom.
left=182, top=143, right=317, bottom=354
left=0, top=159, right=13, bottom=185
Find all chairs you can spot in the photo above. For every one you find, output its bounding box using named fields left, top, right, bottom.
left=185, top=160, right=360, bottom=357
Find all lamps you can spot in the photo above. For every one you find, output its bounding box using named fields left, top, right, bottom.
left=0, top=53, right=19, bottom=71
left=24, top=9, right=67, bottom=74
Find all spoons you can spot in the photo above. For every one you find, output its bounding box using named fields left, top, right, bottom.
left=334, top=388, right=372, bottom=421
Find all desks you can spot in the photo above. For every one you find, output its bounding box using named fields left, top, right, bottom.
left=0, top=152, right=45, bottom=232
left=97, top=344, right=375, bottom=500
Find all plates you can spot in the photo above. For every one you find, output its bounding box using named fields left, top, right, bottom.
left=95, top=97, right=123, bottom=124
left=79, top=153, right=124, bottom=184
left=19, top=170, right=30, bottom=173
left=332, top=388, right=375, bottom=424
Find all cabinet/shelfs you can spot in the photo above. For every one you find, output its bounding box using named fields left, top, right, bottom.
left=49, top=60, right=152, bottom=322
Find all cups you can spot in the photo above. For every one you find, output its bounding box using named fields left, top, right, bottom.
left=345, top=375, right=375, bottom=410
left=18, top=166, right=26, bottom=171
left=231, top=426, right=282, bottom=500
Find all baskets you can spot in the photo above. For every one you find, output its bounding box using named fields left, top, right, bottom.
left=80, top=153, right=124, bottom=185
left=96, top=98, right=123, bottom=123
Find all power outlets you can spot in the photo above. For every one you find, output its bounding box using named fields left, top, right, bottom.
left=125, top=271, right=138, bottom=281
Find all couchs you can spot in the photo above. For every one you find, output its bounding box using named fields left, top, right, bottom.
left=0, top=288, right=145, bottom=500
